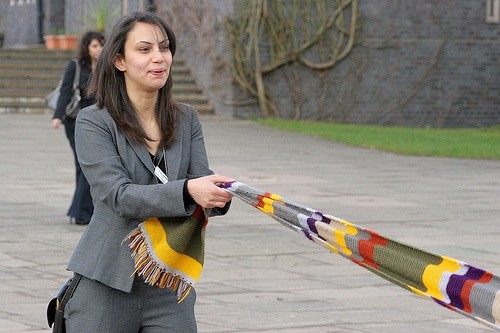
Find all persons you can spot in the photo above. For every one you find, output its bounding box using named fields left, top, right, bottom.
left=56, top=9, right=232, bottom=333
left=50, top=29, right=110, bottom=227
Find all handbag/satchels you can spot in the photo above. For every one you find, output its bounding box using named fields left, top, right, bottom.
left=46, top=278, right=74, bottom=332
left=42, top=58, right=80, bottom=119
